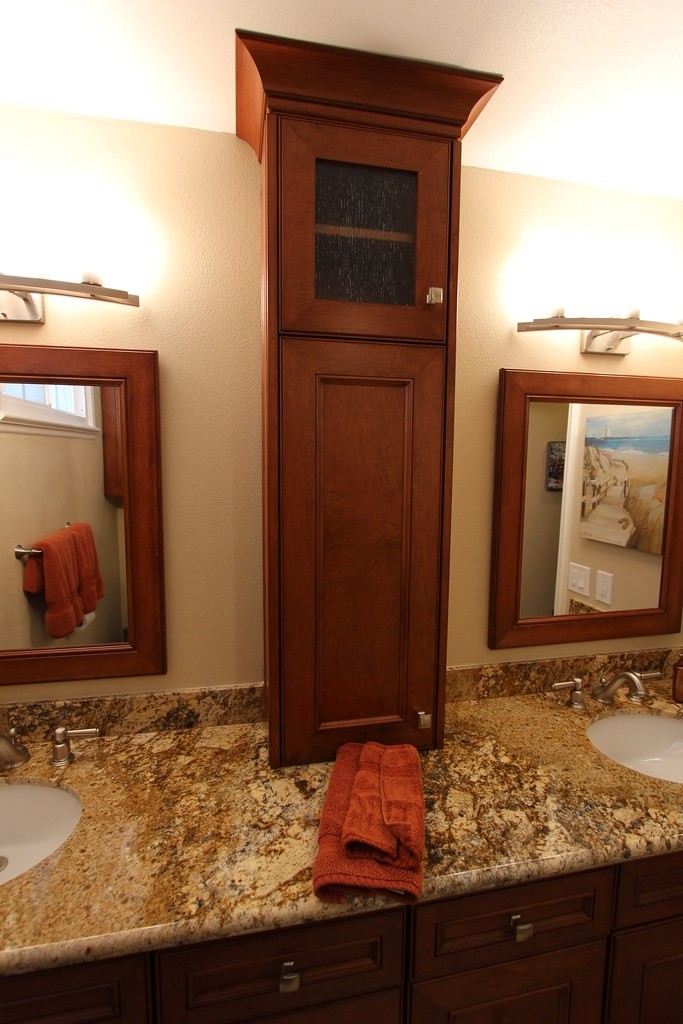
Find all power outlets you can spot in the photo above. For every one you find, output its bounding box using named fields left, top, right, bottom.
left=595, top=570, right=614, bottom=604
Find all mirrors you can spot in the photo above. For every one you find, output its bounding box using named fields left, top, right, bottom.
left=0, top=344, right=167, bottom=686
left=488, top=368, right=683, bottom=650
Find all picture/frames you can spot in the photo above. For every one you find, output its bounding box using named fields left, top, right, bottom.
left=545, top=441, right=565, bottom=492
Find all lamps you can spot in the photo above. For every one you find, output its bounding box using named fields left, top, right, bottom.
left=514, top=307, right=683, bottom=357
left=0, top=274, right=139, bottom=323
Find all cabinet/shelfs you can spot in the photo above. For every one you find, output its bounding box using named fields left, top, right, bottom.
left=235, top=28, right=503, bottom=769
left=0, top=843, right=683, bottom=1024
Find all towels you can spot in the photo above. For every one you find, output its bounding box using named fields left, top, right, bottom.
left=311, top=741, right=425, bottom=904
left=23, top=533, right=84, bottom=638
left=57, top=522, right=104, bottom=614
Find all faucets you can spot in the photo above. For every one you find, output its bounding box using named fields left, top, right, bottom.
left=591, top=672, right=647, bottom=705
left=0, top=727, right=32, bottom=770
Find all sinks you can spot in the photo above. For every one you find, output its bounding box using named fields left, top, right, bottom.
left=585, top=709, right=683, bottom=784
left=0, top=776, right=83, bottom=886
